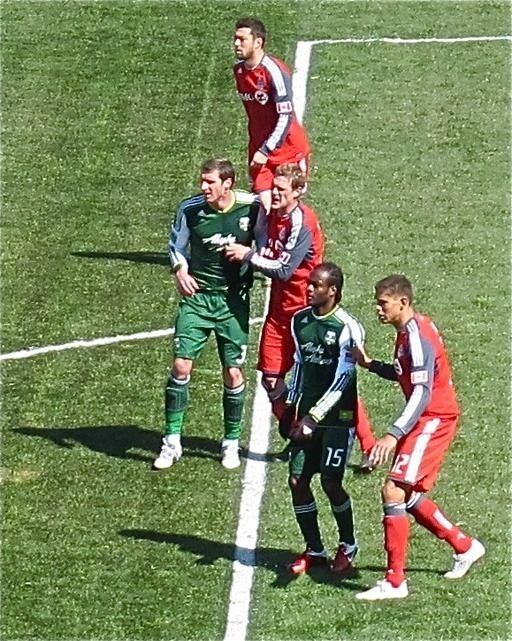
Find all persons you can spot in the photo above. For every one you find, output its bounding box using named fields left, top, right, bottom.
left=224, top=160, right=382, bottom=471
left=150, top=155, right=269, bottom=474
left=278, top=261, right=366, bottom=576
left=231, top=17, right=311, bottom=213
left=353, top=272, right=487, bottom=600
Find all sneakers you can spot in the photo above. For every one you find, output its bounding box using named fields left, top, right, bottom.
left=221, top=438, right=241, bottom=470
left=444, top=537, right=486, bottom=580
left=354, top=580, right=409, bottom=600
left=153, top=433, right=184, bottom=468
left=331, top=539, right=358, bottom=574
left=290, top=545, right=327, bottom=575
